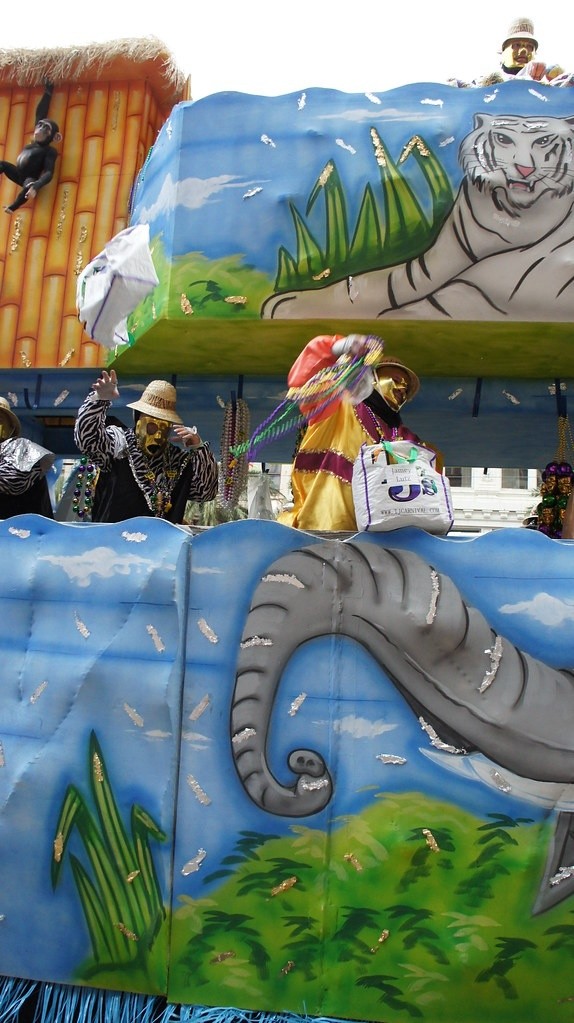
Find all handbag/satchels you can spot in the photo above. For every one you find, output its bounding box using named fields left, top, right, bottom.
left=75, top=225, right=159, bottom=347
left=352, top=440, right=454, bottom=535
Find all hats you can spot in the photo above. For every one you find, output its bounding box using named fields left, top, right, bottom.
left=379, top=357, right=420, bottom=402
left=0, top=397, right=20, bottom=438
left=502, top=18, right=538, bottom=51
left=126, top=379, right=184, bottom=424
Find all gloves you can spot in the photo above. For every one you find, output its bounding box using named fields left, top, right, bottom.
left=342, top=333, right=384, bottom=365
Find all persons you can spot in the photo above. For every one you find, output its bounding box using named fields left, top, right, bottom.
left=490, top=15, right=539, bottom=81
left=0, top=396, right=56, bottom=519
left=74, top=370, right=219, bottom=525
left=277, top=333, right=442, bottom=533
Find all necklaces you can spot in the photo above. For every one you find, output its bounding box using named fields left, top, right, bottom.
left=143, top=452, right=171, bottom=519
left=229, top=335, right=384, bottom=464
left=72, top=456, right=94, bottom=518
left=220, top=399, right=251, bottom=510
